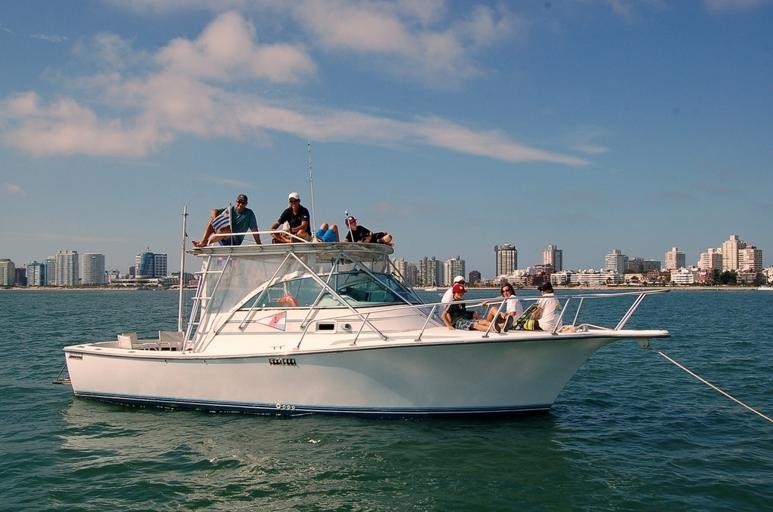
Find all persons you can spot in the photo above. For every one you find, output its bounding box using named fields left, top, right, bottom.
left=272, top=192, right=392, bottom=244
left=440, top=275, right=563, bottom=334
left=192, top=195, right=261, bottom=247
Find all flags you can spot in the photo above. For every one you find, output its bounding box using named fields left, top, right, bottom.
left=210, top=206, right=230, bottom=233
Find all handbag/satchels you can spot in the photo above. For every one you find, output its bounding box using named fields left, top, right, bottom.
left=523, top=313, right=535, bottom=331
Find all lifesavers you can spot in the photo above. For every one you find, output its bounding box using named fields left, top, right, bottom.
left=277, top=294, right=298, bottom=308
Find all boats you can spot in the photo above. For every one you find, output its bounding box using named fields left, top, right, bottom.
left=59, top=133, right=674, bottom=417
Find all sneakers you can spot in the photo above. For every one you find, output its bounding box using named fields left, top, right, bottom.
left=493, top=315, right=513, bottom=333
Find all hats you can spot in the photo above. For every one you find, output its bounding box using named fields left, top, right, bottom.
left=345, top=216, right=357, bottom=225
left=289, top=192, right=299, bottom=200
left=238, top=194, right=248, bottom=203
left=453, top=284, right=467, bottom=293
left=454, top=276, right=465, bottom=283
left=538, top=281, right=552, bottom=289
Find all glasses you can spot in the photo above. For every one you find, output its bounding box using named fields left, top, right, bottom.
left=539, top=288, right=546, bottom=292
left=502, top=288, right=511, bottom=293
